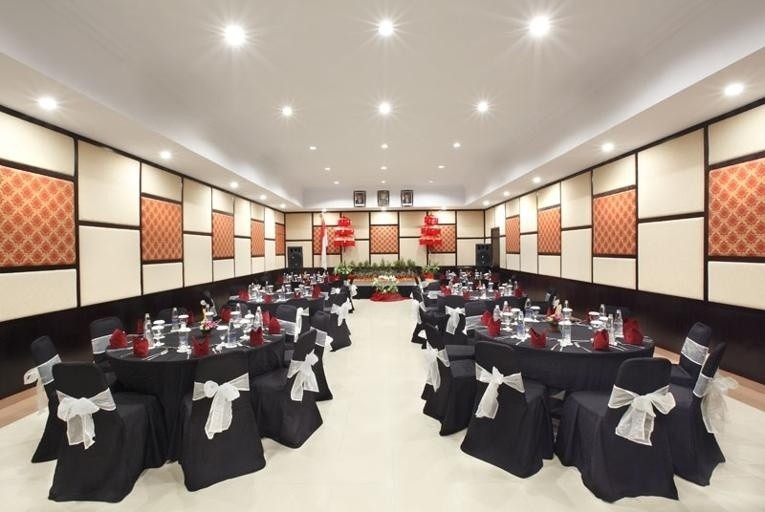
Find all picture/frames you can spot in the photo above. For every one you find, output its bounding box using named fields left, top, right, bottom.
left=353, top=191, right=366, bottom=208
left=400, top=190, right=413, bottom=207
left=377, top=190, right=389, bottom=207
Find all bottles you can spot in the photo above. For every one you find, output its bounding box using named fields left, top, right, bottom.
left=446, top=266, right=623, bottom=348
left=142, top=271, right=329, bottom=354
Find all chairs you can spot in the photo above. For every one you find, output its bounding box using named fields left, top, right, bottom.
left=165, top=350, right=267, bottom=493
left=411, top=267, right=655, bottom=420
left=665, top=340, right=730, bottom=487
left=551, top=358, right=683, bottom=504
left=48, top=360, right=166, bottom=503
left=461, top=339, right=556, bottom=480
left=88, top=270, right=357, bottom=426
left=249, top=329, right=324, bottom=450
left=421, top=320, right=477, bottom=438
left=669, top=320, right=714, bottom=388
left=29, top=337, right=63, bottom=463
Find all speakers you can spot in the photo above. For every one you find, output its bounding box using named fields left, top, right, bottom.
left=288, top=247, right=303, bottom=275
left=476, top=244, right=492, bottom=274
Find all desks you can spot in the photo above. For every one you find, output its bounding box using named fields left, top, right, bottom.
left=351, top=277, right=418, bottom=298
